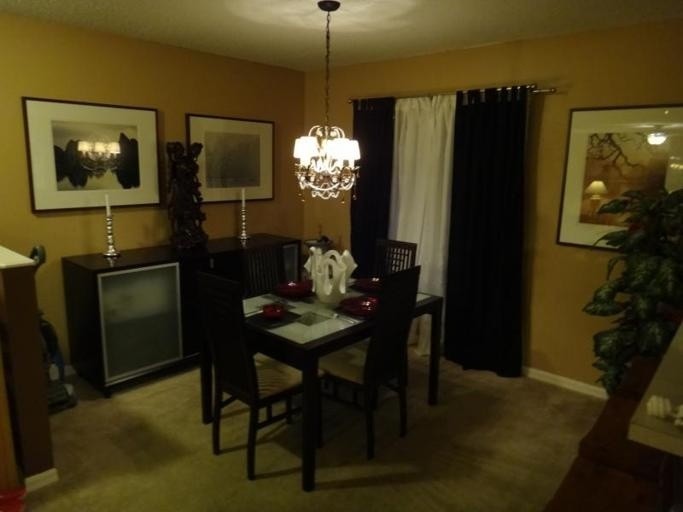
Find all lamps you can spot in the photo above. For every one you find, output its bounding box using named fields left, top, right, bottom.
left=585, top=181, right=607, bottom=217
left=292, top=0, right=362, bottom=205
left=76, top=125, right=121, bottom=176
left=647, top=395, right=683, bottom=426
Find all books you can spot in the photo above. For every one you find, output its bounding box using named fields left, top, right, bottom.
left=242, top=292, right=288, bottom=318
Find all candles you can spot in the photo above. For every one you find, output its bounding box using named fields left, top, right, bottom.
left=104, top=192, right=111, bottom=218
left=241, top=186, right=246, bottom=209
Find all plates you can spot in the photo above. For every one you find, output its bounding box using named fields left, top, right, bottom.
left=262, top=303, right=284, bottom=319
left=278, top=280, right=311, bottom=298
left=344, top=295, right=380, bottom=316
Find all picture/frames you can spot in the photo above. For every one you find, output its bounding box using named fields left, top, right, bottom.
left=21, top=96, right=162, bottom=213
left=184, top=112, right=275, bottom=203
left=554, top=102, right=683, bottom=250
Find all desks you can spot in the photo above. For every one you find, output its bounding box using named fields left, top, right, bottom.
left=540, top=300, right=683, bottom=511
left=0, top=244, right=58, bottom=496
left=61, top=233, right=301, bottom=397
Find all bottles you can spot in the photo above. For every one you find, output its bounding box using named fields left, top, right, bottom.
left=369, top=273, right=381, bottom=286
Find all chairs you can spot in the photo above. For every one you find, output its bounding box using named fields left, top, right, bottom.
left=318, top=265, right=421, bottom=460
left=192, top=270, right=326, bottom=480
left=243, top=241, right=287, bottom=297
left=373, top=239, right=418, bottom=277
